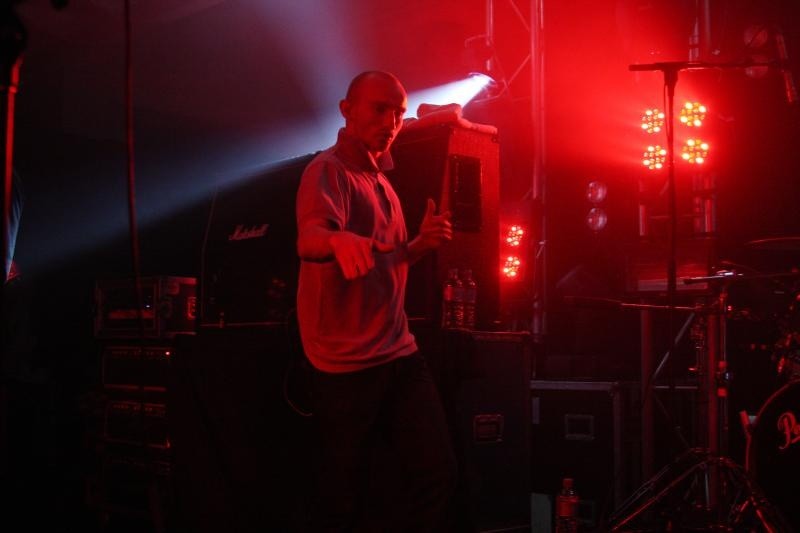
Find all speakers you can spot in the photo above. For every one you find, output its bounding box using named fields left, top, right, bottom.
left=200, top=126, right=503, bottom=326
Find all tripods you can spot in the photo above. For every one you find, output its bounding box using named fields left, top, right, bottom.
left=563, top=270, right=748, bottom=533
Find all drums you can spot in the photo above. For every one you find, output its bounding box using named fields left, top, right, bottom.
left=745, top=380, right=800, bottom=533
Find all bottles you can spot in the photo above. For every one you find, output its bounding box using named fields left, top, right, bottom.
left=441, top=269, right=479, bottom=328
left=555, top=477, right=579, bottom=533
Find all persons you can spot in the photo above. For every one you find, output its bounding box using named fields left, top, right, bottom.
left=295, top=69, right=455, bottom=530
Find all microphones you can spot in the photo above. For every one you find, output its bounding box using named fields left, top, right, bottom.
left=775, top=30, right=799, bottom=104
